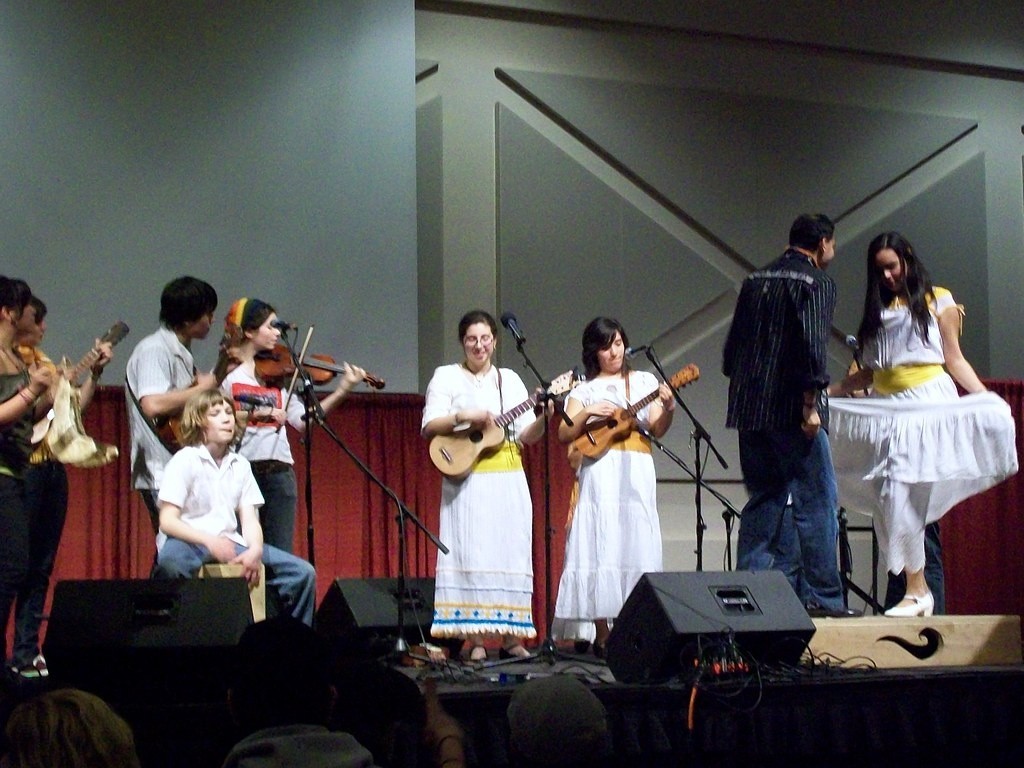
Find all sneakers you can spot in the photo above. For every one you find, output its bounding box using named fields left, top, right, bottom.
left=11, top=654, right=48, bottom=676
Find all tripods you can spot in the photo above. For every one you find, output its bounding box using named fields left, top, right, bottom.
left=471, top=343, right=609, bottom=671
left=312, top=414, right=474, bottom=675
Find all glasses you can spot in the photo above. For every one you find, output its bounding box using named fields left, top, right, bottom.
left=465, top=333, right=494, bottom=346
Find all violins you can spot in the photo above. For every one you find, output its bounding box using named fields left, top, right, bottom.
left=255, top=342, right=386, bottom=391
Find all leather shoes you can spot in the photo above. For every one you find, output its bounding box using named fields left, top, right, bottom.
left=469, top=644, right=525, bottom=661
left=804, top=599, right=863, bottom=617
left=594, top=638, right=608, bottom=659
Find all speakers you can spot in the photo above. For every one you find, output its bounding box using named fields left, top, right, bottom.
left=603, top=569, right=817, bottom=684
left=312, top=577, right=465, bottom=660
left=42, top=578, right=255, bottom=676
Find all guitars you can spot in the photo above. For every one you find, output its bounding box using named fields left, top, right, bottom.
left=428, top=367, right=582, bottom=483
left=155, top=320, right=244, bottom=457
left=572, top=361, right=701, bottom=462
left=30, top=319, right=130, bottom=467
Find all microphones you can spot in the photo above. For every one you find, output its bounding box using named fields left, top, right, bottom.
left=269, top=319, right=296, bottom=330
left=845, top=335, right=863, bottom=360
left=234, top=395, right=272, bottom=407
left=625, top=345, right=648, bottom=360
left=500, top=312, right=527, bottom=344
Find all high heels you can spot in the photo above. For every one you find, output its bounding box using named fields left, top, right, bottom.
left=884, top=590, right=934, bottom=617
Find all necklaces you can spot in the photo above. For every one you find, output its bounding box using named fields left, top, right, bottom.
left=465, top=361, right=480, bottom=382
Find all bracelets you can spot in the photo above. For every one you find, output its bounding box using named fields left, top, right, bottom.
left=89, top=364, right=103, bottom=381
left=455, top=413, right=461, bottom=424
left=19, top=387, right=39, bottom=406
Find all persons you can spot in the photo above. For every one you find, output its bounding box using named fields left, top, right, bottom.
left=125, top=276, right=366, bottom=578
left=551, top=317, right=677, bottom=659
left=421, top=310, right=555, bottom=663
left=156, top=388, right=316, bottom=628
left=0, top=615, right=609, bottom=768
left=0, top=275, right=111, bottom=677
left=721, top=214, right=1019, bottom=619
